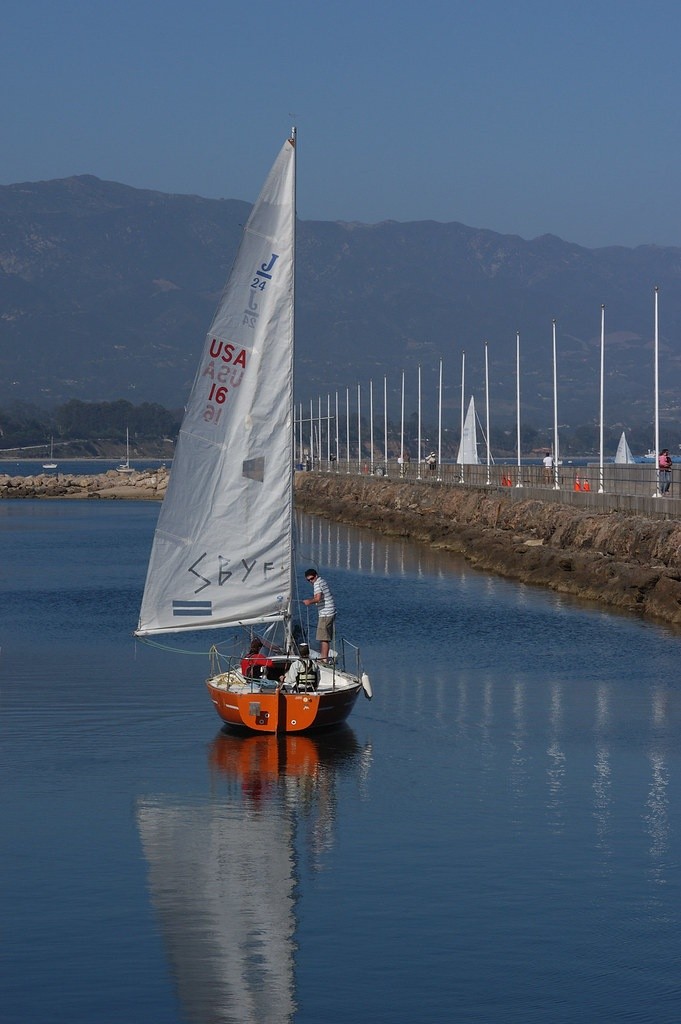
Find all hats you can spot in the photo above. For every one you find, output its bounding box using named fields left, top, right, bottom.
left=250, top=639, right=263, bottom=648
left=299, top=643, right=309, bottom=652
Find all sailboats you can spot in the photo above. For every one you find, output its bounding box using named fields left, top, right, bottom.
left=42, top=435, right=58, bottom=469
left=116, top=427, right=135, bottom=473
left=130, top=121, right=371, bottom=734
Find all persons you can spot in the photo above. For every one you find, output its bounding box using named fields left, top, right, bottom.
left=331, top=453, right=337, bottom=464
left=659, top=450, right=671, bottom=472
left=427, top=453, right=437, bottom=476
left=658, top=449, right=671, bottom=497
left=543, top=453, right=553, bottom=486
left=425, top=451, right=434, bottom=461
left=241, top=638, right=280, bottom=687
left=284, top=644, right=321, bottom=693
left=302, top=569, right=337, bottom=664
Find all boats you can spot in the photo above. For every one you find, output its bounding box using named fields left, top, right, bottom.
left=645, top=450, right=657, bottom=459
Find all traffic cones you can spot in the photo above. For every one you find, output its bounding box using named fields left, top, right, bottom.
left=506, top=471, right=512, bottom=487
left=501, top=471, right=507, bottom=486
left=574, top=474, right=582, bottom=491
left=582, top=475, right=590, bottom=492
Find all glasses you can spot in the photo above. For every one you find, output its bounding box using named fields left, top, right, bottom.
left=307, top=576, right=315, bottom=581
